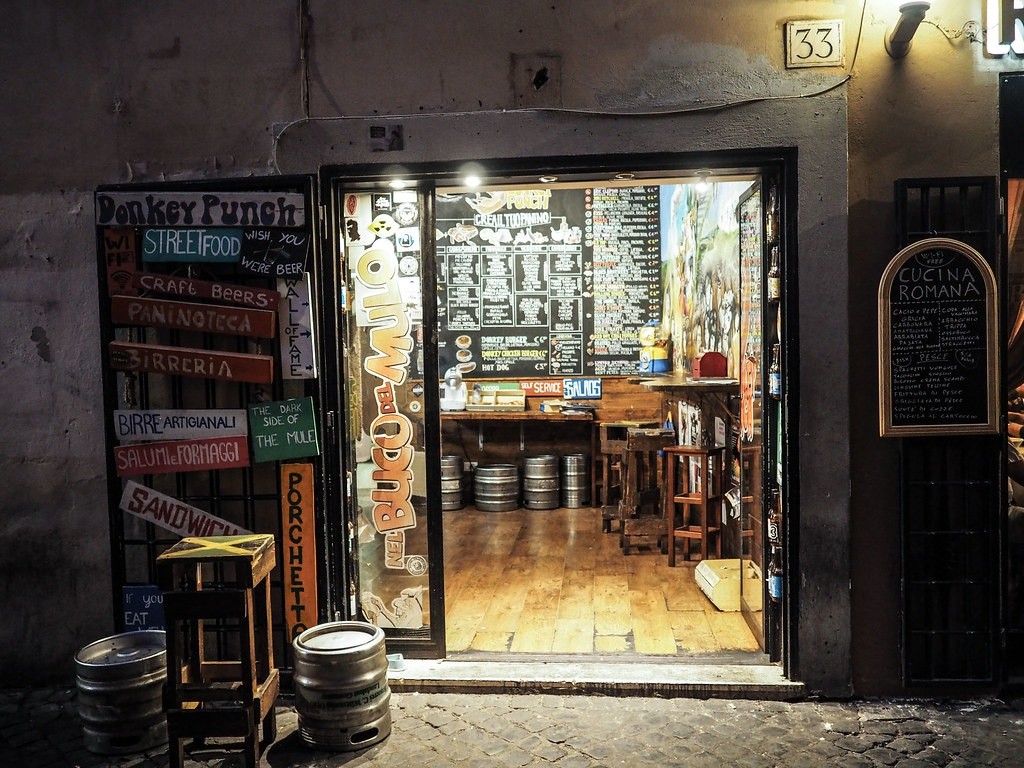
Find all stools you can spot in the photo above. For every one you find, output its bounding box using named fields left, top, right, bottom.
left=158, top=534, right=280, bottom=768
left=598, top=421, right=660, bottom=534
left=664, top=445, right=728, bottom=566
left=619, top=428, right=676, bottom=556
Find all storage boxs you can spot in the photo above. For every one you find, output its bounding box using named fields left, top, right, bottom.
left=466, top=390, right=526, bottom=411
left=692, top=352, right=727, bottom=378
left=539, top=403, right=560, bottom=413
left=694, top=559, right=762, bottom=613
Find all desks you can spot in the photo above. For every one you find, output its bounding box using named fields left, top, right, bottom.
left=631, top=370, right=740, bottom=429
left=440, top=412, right=597, bottom=507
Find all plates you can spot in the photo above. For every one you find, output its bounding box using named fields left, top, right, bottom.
left=456, top=362, right=476, bottom=373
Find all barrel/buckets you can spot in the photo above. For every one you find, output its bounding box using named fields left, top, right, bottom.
left=441, top=456, right=464, bottom=510
left=474, top=464, right=520, bottom=512
left=563, top=454, right=588, bottom=509
left=524, top=455, right=560, bottom=509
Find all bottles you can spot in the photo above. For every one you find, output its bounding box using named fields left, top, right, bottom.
left=766, top=211, right=775, bottom=244
left=768, top=547, right=781, bottom=603
left=473, top=382, right=481, bottom=403
left=768, top=348, right=781, bottom=400
left=768, top=247, right=780, bottom=302
left=767, top=489, right=781, bottom=543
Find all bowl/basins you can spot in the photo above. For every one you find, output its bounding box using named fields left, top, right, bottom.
left=455, top=335, right=472, bottom=349
left=456, top=349, right=473, bottom=363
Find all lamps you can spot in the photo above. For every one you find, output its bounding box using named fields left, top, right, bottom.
left=884, top=0, right=931, bottom=59
